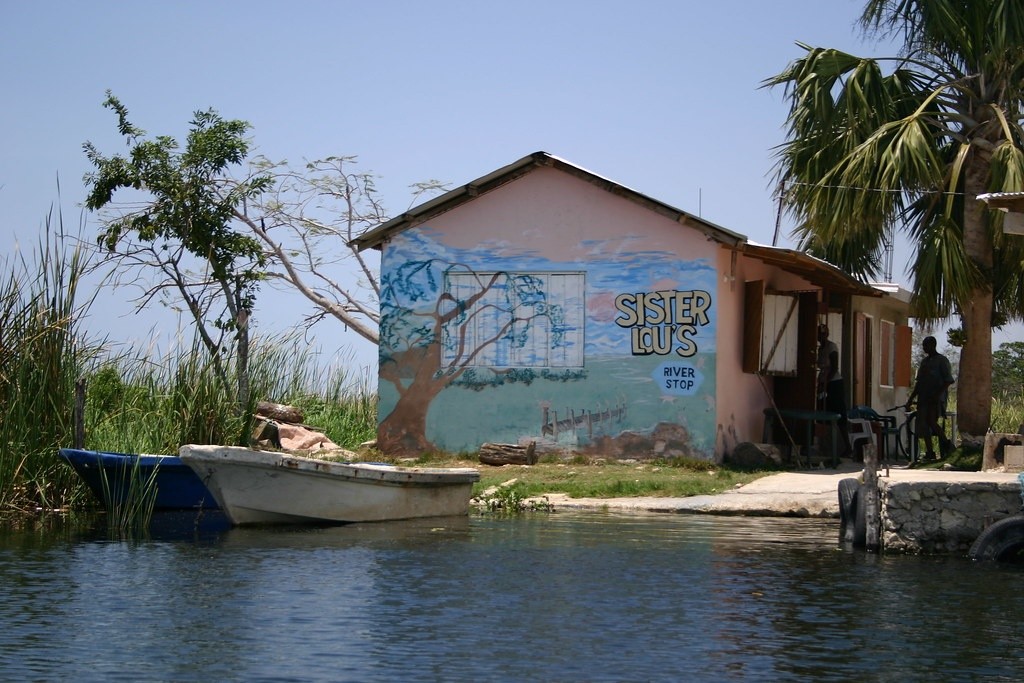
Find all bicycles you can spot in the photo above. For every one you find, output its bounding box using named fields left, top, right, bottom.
left=886, top=401, right=921, bottom=461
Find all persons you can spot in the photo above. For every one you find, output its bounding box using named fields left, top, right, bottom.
left=905, top=336, right=955, bottom=461
left=817, top=322, right=856, bottom=459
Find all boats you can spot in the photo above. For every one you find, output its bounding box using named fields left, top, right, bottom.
left=55, top=447, right=240, bottom=538
left=177, top=443, right=483, bottom=528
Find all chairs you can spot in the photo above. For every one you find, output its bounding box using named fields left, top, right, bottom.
left=845, top=402, right=901, bottom=463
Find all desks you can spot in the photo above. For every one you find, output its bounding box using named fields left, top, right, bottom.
left=762, top=407, right=842, bottom=469
left=904, top=411, right=957, bottom=463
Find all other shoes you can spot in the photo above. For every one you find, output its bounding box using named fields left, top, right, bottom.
left=923, top=451, right=936, bottom=461
left=940, top=444, right=956, bottom=460
